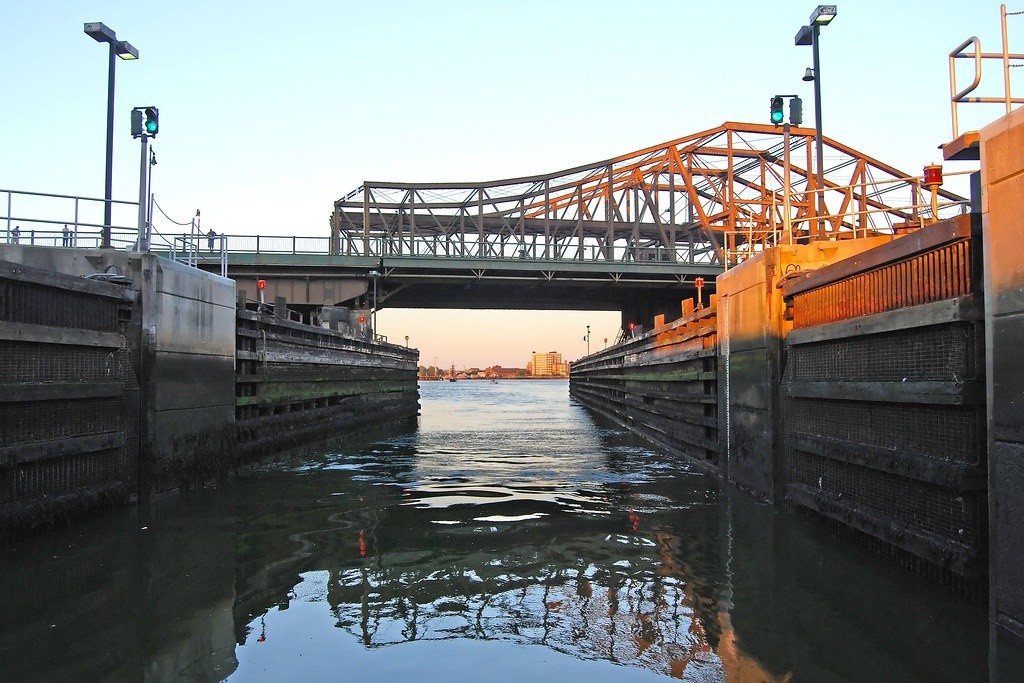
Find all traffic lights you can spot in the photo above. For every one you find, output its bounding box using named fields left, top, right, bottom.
left=145, top=107, right=160, bottom=133
left=770, top=96, right=784, bottom=124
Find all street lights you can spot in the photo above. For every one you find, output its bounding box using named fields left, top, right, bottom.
left=587, top=325, right=590, bottom=355
left=83, top=22, right=140, bottom=249
left=368, top=271, right=380, bottom=341
left=793, top=4, right=837, bottom=240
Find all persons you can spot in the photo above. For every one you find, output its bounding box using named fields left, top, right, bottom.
left=100, top=227, right=105, bottom=246
left=62, top=225, right=69, bottom=247
left=207, top=228, right=216, bottom=252
left=11, top=226, right=21, bottom=244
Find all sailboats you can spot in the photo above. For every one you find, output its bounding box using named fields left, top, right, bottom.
left=449, top=364, right=457, bottom=382
